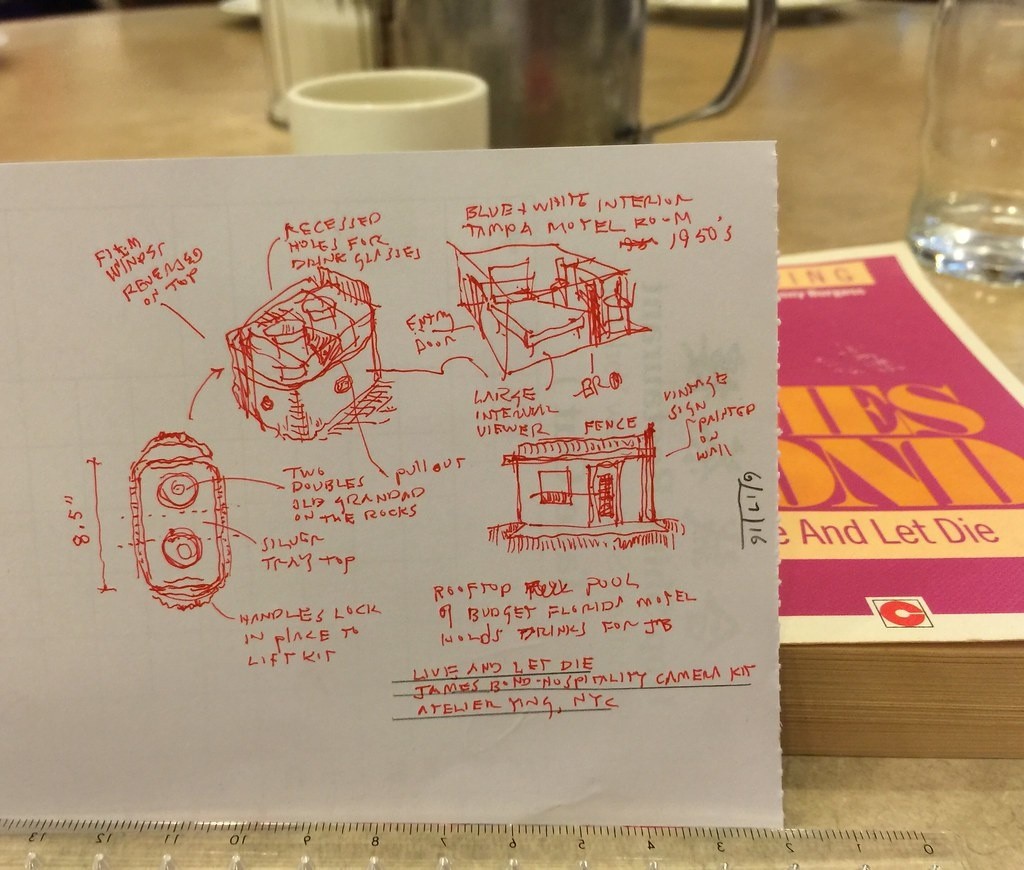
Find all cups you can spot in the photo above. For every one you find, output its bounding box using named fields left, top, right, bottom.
left=285, top=69, right=491, bottom=153
left=256, top=0, right=381, bottom=127
left=906, top=0, right=1024, bottom=289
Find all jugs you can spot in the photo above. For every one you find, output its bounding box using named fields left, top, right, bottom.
left=369, top=0, right=779, bottom=150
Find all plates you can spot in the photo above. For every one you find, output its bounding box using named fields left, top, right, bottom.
left=220, top=0, right=260, bottom=19
